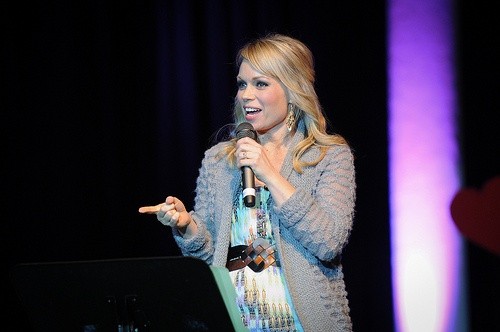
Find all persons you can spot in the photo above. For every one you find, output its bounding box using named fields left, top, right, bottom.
left=139, top=33, right=357, bottom=332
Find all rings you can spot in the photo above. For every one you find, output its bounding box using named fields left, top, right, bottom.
left=243, top=152, right=247, bottom=159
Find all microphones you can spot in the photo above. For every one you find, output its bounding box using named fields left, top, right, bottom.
left=235, top=122, right=258, bottom=207
